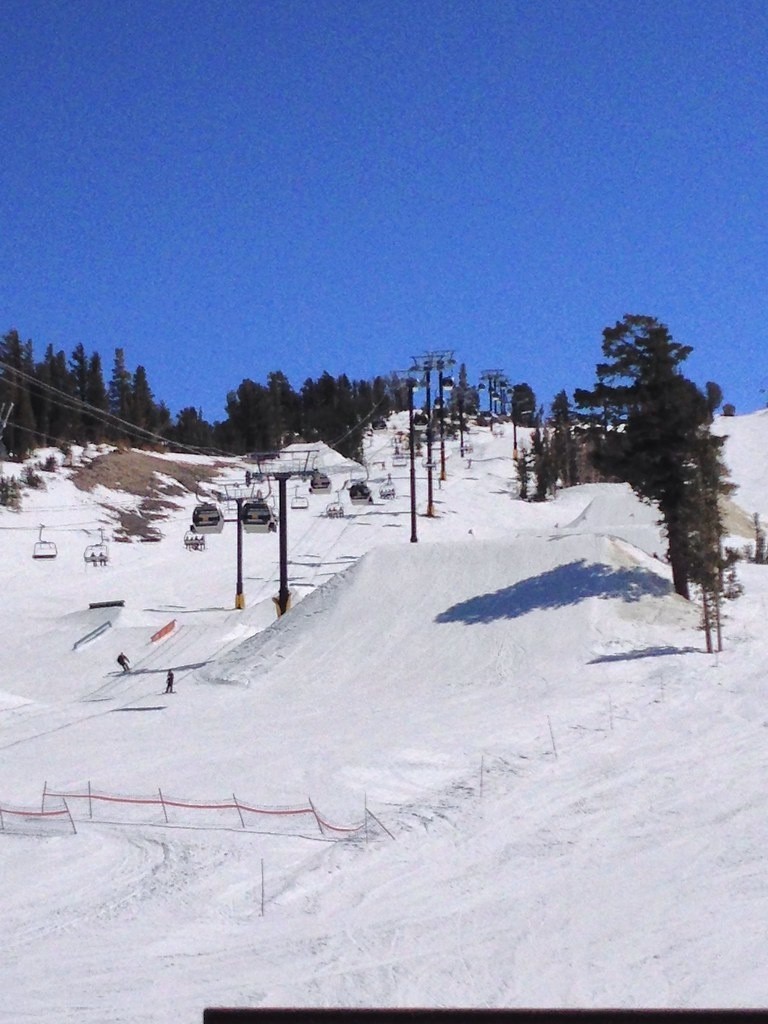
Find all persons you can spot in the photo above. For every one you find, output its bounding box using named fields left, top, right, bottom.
left=380, top=488, right=395, bottom=499
left=98, top=552, right=107, bottom=566
left=165, top=669, right=174, bottom=693
left=184, top=535, right=205, bottom=552
left=328, top=505, right=345, bottom=519
left=90, top=552, right=97, bottom=567
left=118, top=652, right=131, bottom=670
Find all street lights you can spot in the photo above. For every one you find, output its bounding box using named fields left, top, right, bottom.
left=477, top=369, right=514, bottom=432
left=406, top=349, right=457, bottom=544
